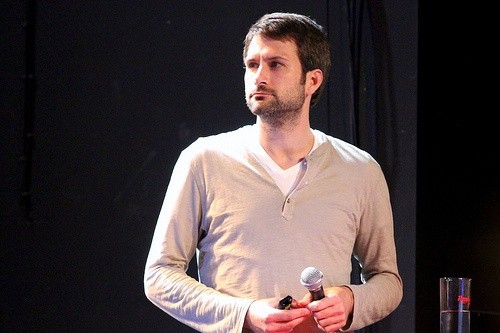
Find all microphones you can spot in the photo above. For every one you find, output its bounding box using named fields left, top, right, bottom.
left=300, top=267, right=325, bottom=301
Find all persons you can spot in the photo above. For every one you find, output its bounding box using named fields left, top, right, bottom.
left=143, top=10, right=403, bottom=333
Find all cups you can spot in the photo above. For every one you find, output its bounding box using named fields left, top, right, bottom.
left=440, top=278, right=471, bottom=333
